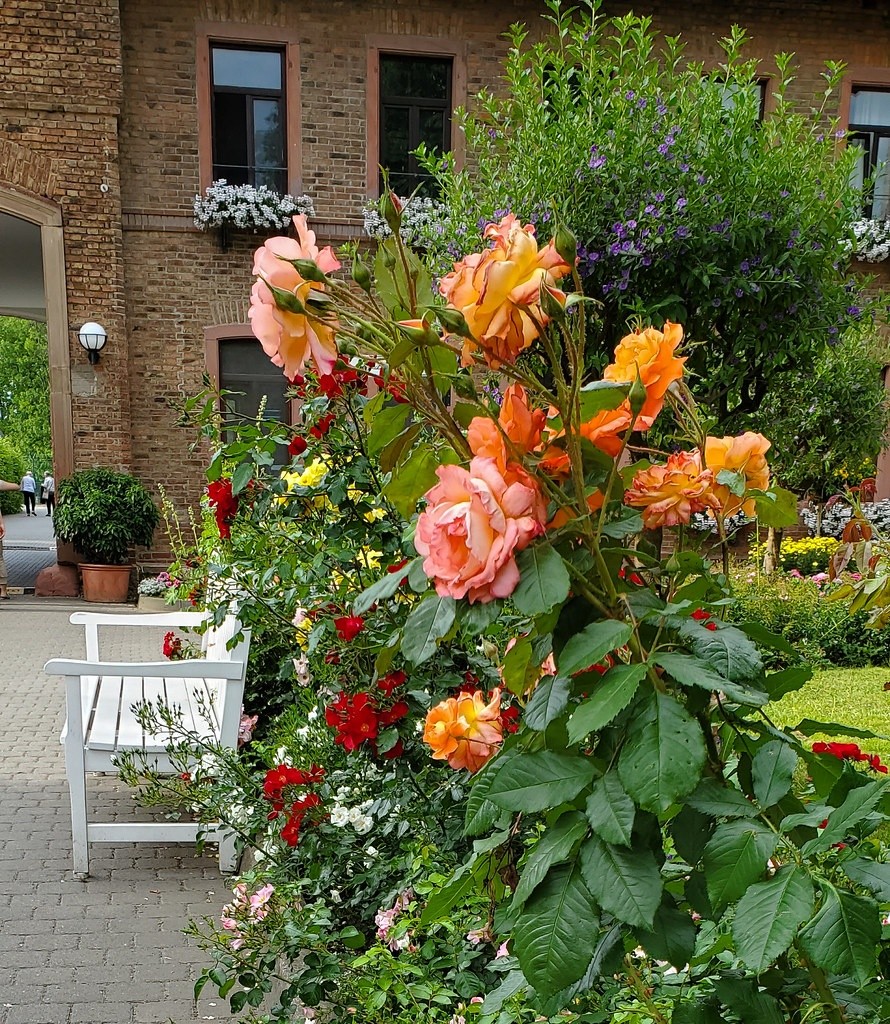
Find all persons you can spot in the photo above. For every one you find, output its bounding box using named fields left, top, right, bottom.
left=41, top=471, right=55, bottom=516
left=21, top=471, right=37, bottom=516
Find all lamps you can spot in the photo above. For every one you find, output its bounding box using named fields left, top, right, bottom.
left=78, top=322, right=108, bottom=365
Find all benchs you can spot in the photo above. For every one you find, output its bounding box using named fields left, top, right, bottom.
left=43, top=555, right=252, bottom=880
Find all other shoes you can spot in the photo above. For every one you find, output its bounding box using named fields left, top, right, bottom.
left=45, top=514, right=51, bottom=516
left=27, top=514, right=30, bottom=517
left=31, top=512, right=37, bottom=516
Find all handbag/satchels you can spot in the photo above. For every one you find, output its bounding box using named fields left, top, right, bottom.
left=42, top=491, right=49, bottom=499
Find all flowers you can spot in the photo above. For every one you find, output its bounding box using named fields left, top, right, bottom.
left=840, top=218, right=890, bottom=262
left=364, top=195, right=451, bottom=246
left=193, top=179, right=315, bottom=233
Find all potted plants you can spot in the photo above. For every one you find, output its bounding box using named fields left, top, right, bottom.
left=52, top=467, right=160, bottom=603
